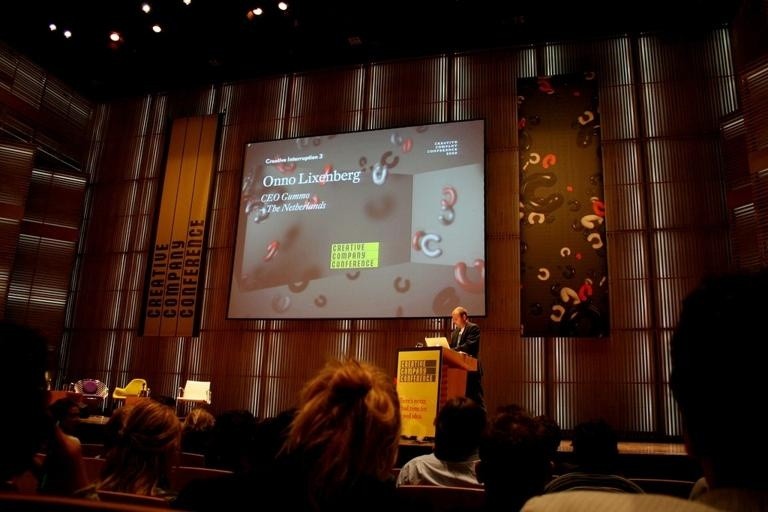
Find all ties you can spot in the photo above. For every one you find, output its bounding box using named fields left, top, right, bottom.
left=456, top=331, right=463, bottom=347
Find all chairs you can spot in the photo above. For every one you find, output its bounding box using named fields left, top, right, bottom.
left=0, top=377, right=696, bottom=511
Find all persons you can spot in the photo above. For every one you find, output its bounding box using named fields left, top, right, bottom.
left=205, top=359, right=487, bottom=512
left=671, top=271, right=767, bottom=512
left=2, top=320, right=215, bottom=498
left=475, top=404, right=622, bottom=512
left=417, top=306, right=485, bottom=409
